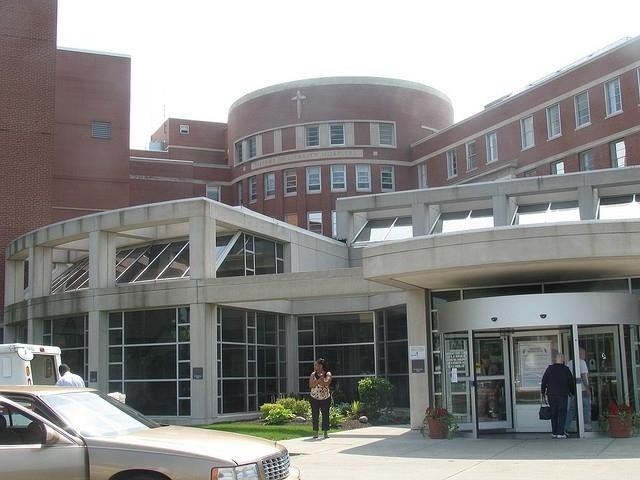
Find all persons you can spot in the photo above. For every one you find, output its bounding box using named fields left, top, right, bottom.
left=308, top=358, right=332, bottom=438
left=564, top=347, right=593, bottom=432
left=480, top=350, right=501, bottom=418
left=540, top=354, right=573, bottom=438
left=55, top=363, right=86, bottom=387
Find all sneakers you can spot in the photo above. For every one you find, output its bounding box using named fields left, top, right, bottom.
left=313, top=434, right=330, bottom=438
left=551, top=431, right=568, bottom=439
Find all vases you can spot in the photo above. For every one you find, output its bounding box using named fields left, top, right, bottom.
left=427, top=418, right=449, bottom=439
left=606, top=415, right=632, bottom=438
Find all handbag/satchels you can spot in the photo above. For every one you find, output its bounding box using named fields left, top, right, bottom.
left=539, top=393, right=552, bottom=420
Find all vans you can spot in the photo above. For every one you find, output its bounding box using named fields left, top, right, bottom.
left=0, top=343, right=61, bottom=385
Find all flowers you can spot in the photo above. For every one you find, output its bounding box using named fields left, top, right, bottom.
left=419, top=406, right=459, bottom=440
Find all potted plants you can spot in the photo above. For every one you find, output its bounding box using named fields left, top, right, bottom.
left=598, top=403, right=640, bottom=430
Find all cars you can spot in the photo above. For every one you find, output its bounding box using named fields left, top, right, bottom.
left=0, top=385, right=290, bottom=480
